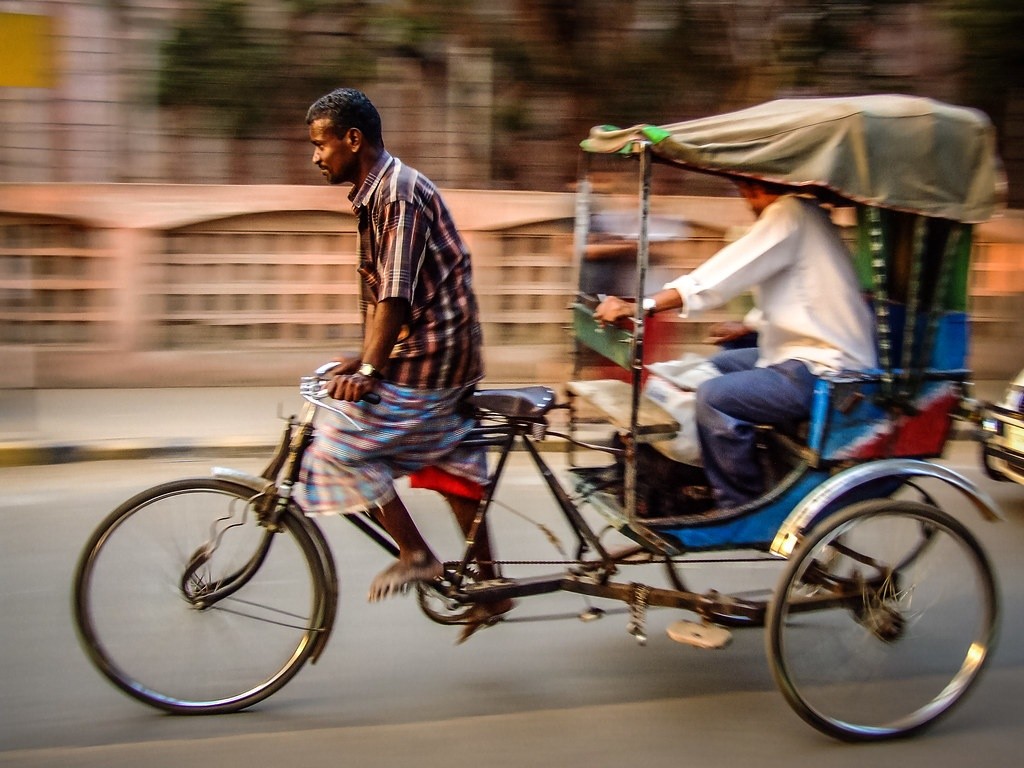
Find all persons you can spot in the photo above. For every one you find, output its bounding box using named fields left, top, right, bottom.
left=298, top=87, right=516, bottom=646
left=596, top=174, right=875, bottom=508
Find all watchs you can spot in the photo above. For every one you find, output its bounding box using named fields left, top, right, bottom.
left=358, top=363, right=378, bottom=377
left=642, top=298, right=656, bottom=318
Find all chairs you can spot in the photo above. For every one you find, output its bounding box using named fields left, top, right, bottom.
left=717, top=294, right=966, bottom=460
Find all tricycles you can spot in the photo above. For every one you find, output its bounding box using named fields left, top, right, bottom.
left=76, top=89, right=1001, bottom=740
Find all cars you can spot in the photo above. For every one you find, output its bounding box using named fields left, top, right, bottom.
left=984, top=366, right=1024, bottom=490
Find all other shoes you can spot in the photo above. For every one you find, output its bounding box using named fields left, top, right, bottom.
left=683, top=485, right=715, bottom=503
